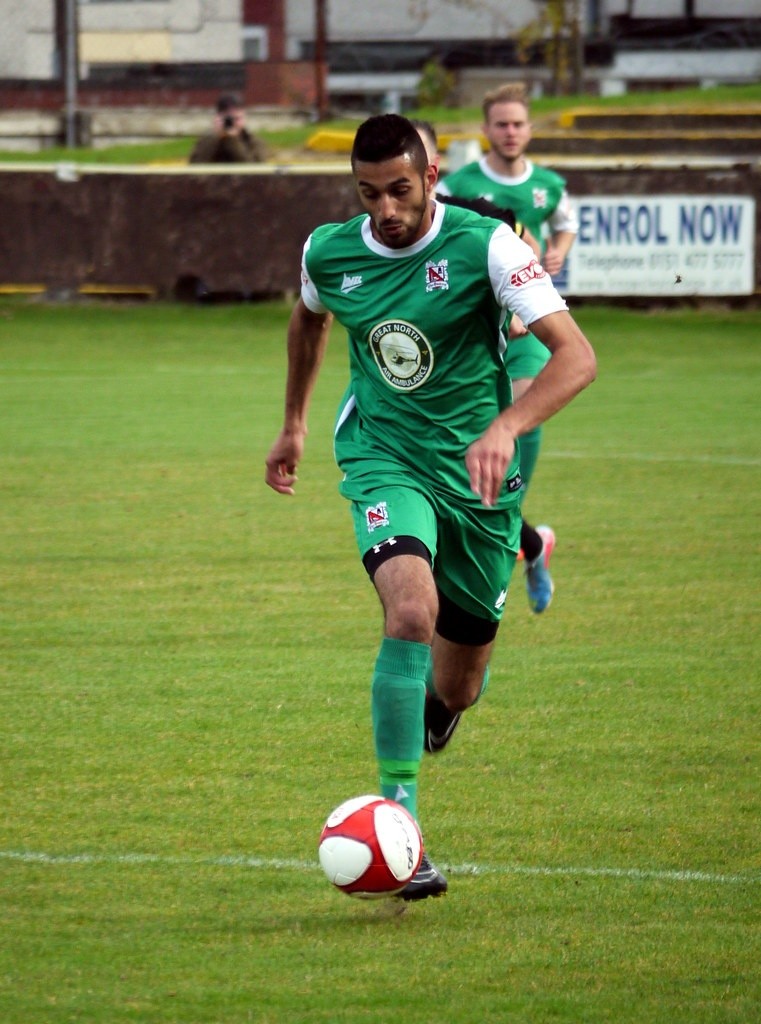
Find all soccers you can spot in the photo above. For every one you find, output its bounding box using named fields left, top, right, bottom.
left=318, top=793, right=425, bottom=903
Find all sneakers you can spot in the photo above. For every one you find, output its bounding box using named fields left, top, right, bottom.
left=392, top=848, right=449, bottom=902
left=423, top=689, right=464, bottom=753
left=522, top=525, right=556, bottom=614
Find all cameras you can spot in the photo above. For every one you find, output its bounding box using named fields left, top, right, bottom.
left=222, top=116, right=234, bottom=129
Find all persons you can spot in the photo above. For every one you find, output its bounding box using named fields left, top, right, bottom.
left=266, top=113, right=598, bottom=902
left=187, top=98, right=264, bottom=163
left=410, top=119, right=556, bottom=614
left=435, top=84, right=578, bottom=504
